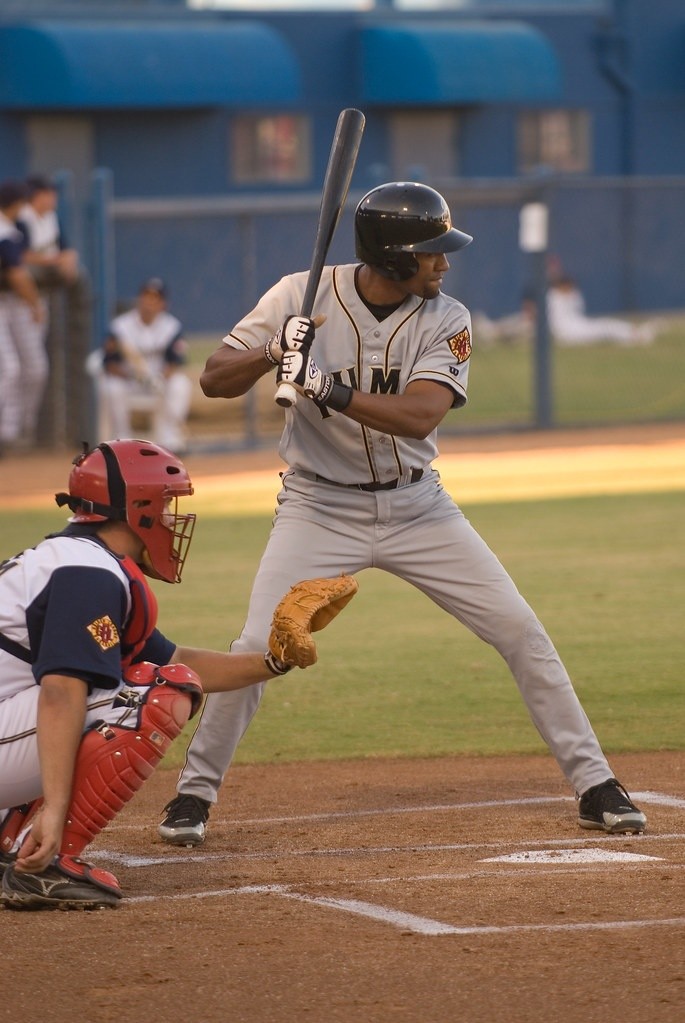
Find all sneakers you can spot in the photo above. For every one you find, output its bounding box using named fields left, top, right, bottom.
left=576, top=778, right=649, bottom=836
left=157, top=794, right=213, bottom=847
left=0, top=852, right=120, bottom=909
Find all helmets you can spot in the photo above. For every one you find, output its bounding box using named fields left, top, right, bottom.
left=354, top=181, right=475, bottom=282
left=66, top=440, right=196, bottom=583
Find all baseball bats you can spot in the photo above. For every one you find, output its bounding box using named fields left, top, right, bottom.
left=274, top=109, right=368, bottom=409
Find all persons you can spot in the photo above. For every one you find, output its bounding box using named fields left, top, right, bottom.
left=0, top=175, right=81, bottom=458
left=0, top=437, right=313, bottom=912
left=157, top=177, right=652, bottom=847
left=84, top=276, right=197, bottom=462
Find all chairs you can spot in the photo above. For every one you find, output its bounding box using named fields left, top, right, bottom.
left=84, top=349, right=190, bottom=450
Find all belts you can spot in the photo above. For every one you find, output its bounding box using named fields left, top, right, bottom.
left=315, top=469, right=423, bottom=493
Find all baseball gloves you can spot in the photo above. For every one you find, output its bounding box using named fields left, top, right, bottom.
left=267, top=573, right=359, bottom=669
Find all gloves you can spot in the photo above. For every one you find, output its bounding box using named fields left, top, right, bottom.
left=263, top=315, right=354, bottom=419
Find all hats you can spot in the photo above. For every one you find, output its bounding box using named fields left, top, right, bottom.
left=25, top=171, right=58, bottom=196
left=141, top=275, right=168, bottom=300
left=0, top=177, right=30, bottom=209
